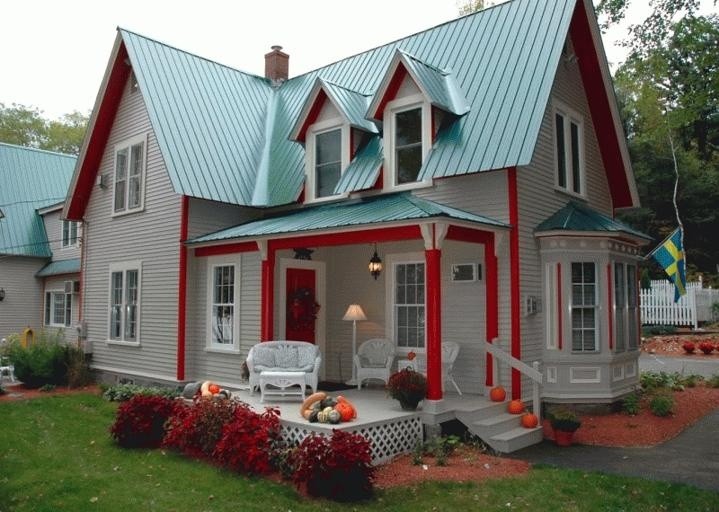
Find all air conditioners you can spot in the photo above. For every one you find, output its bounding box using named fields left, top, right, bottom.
left=64, top=280, right=80, bottom=295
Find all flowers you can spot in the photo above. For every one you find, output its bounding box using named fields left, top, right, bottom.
left=386, top=370, right=429, bottom=403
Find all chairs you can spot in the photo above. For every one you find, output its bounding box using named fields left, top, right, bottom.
left=353, top=338, right=395, bottom=390
left=422, top=341, right=462, bottom=396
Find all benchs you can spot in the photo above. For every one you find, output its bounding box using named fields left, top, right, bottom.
left=246, top=340, right=322, bottom=397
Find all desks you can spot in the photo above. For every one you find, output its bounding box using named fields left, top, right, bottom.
left=398, top=358, right=418, bottom=375
left=258, top=370, right=306, bottom=402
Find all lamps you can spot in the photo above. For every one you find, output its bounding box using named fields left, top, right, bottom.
left=342, top=304, right=367, bottom=386
left=368, top=242, right=383, bottom=280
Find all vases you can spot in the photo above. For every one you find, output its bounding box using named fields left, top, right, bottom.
left=400, top=398, right=419, bottom=410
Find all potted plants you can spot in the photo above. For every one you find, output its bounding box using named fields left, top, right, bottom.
left=545, top=405, right=580, bottom=446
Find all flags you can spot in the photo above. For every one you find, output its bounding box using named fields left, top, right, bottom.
left=652, top=228, right=688, bottom=302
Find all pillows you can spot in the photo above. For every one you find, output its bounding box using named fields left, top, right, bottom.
left=252, top=343, right=318, bottom=368
left=367, top=347, right=386, bottom=366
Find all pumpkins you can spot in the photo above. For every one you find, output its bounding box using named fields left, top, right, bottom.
left=300, top=392, right=356, bottom=422
left=508, top=399, right=523, bottom=414
left=490, top=387, right=506, bottom=401
left=522, top=413, right=538, bottom=428
left=182, top=382, right=231, bottom=400
